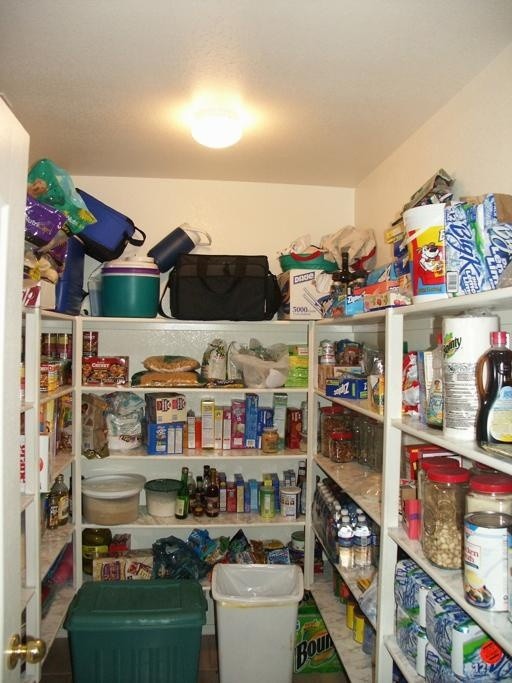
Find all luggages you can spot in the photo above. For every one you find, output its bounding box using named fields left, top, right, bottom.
left=70, top=188, right=146, bottom=263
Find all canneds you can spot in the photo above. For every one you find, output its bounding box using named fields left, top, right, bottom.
left=262, top=428, right=279, bottom=454
left=260, top=486, right=275, bottom=519
left=299, top=402, right=353, bottom=463
left=290, top=530, right=305, bottom=552
left=279, top=486, right=301, bottom=520
left=319, top=340, right=336, bottom=364
left=347, top=594, right=375, bottom=654
left=417, top=458, right=511, bottom=614
left=37, top=330, right=98, bottom=393
left=41, top=493, right=59, bottom=530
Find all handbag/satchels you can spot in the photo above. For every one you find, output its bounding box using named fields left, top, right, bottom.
left=159, top=254, right=281, bottom=321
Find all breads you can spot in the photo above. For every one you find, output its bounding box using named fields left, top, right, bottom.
left=132, top=355, right=204, bottom=388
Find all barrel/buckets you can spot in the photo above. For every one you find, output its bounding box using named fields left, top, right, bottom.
left=102, top=257, right=159, bottom=317
left=400, top=202, right=445, bottom=302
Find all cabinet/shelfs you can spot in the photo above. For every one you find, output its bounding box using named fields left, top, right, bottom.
left=20, top=287, right=511, bottom=683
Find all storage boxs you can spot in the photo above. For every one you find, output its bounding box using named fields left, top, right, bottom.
left=276, top=268, right=322, bottom=319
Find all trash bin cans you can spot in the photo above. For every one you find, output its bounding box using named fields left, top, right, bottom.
left=210, top=563, right=304, bottom=683
left=64, top=578, right=208, bottom=683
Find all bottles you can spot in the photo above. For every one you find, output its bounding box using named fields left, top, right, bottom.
left=465, top=473, right=512, bottom=516
left=347, top=409, right=383, bottom=474
left=337, top=250, right=354, bottom=287
left=51, top=474, right=69, bottom=526
left=419, top=466, right=471, bottom=570
left=328, top=430, right=354, bottom=464
left=174, top=464, right=220, bottom=519
left=46, top=492, right=60, bottom=530
left=311, top=477, right=380, bottom=572
left=320, top=405, right=346, bottom=458
